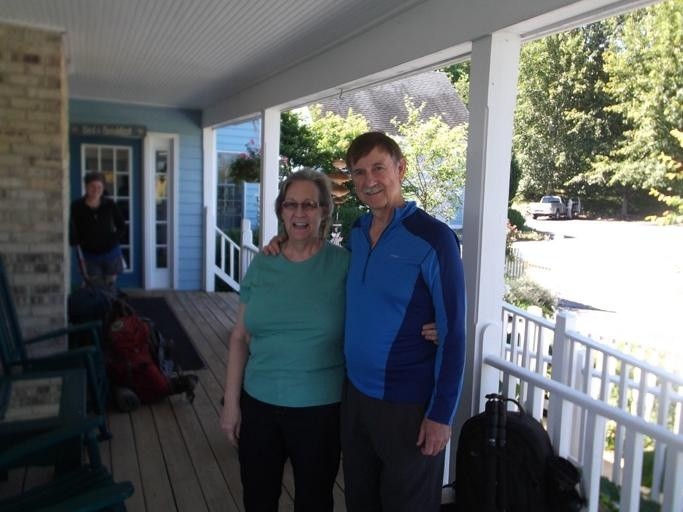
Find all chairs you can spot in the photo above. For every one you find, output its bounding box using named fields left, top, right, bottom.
left=1, top=259, right=135, bottom=511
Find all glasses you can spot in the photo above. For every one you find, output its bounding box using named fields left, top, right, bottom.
left=281, top=198, right=323, bottom=212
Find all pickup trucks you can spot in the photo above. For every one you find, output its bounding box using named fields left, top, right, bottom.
left=527, top=194, right=581, bottom=219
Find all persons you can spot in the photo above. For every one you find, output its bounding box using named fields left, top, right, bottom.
left=263, top=133, right=468, bottom=512
left=219, top=169, right=440, bottom=512
left=69, top=172, right=127, bottom=284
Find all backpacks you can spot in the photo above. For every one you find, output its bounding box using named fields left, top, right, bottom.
left=454, top=393, right=583, bottom=512
left=68, top=276, right=200, bottom=410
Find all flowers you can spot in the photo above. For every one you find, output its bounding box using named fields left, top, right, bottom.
left=229, top=139, right=292, bottom=185
